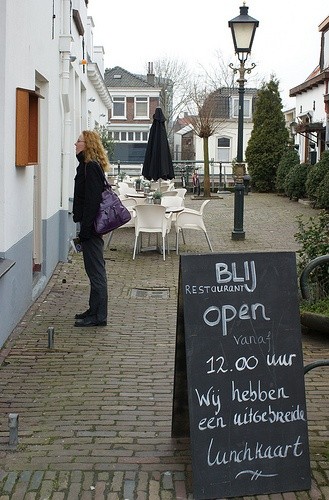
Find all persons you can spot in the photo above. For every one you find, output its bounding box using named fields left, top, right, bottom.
left=72, top=130, right=112, bottom=327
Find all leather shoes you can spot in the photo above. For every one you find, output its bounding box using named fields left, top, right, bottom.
left=74, top=309, right=91, bottom=318
left=74, top=317, right=107, bottom=326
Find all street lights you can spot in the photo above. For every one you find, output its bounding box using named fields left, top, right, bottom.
left=226, top=6, right=259, bottom=240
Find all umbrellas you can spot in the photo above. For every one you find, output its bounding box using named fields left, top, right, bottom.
left=142, top=108, right=175, bottom=194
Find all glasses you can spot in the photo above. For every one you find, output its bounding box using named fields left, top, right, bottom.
left=77, top=140, right=85, bottom=143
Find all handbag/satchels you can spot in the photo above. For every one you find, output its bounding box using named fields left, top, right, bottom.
left=93, top=188, right=131, bottom=234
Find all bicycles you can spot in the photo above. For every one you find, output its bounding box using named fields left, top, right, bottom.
left=175, top=164, right=194, bottom=194
left=189, top=166, right=202, bottom=196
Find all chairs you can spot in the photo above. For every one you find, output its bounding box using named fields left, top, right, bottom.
left=102, top=174, right=213, bottom=262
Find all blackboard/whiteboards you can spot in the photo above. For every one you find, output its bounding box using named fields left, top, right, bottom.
left=171, top=251, right=312, bottom=500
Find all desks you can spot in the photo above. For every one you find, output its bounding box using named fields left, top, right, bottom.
left=117, top=196, right=182, bottom=229
